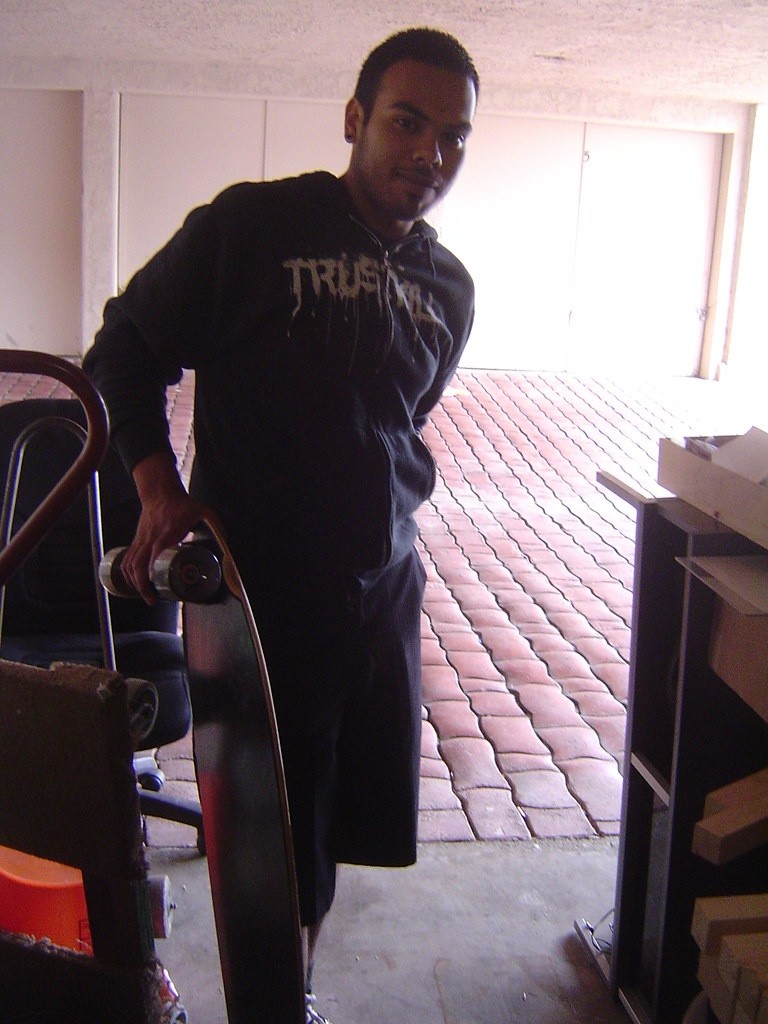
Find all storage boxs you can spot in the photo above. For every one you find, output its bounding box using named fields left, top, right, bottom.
left=656, top=425, right=768, bottom=549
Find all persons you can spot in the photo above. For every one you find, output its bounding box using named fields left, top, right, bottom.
left=81, top=27, right=479, bottom=1023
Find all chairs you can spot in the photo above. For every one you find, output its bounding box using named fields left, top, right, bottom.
left=0, top=397, right=209, bottom=856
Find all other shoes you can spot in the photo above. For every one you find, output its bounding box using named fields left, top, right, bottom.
left=304, top=993, right=332, bottom=1024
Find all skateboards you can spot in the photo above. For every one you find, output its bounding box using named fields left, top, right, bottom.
left=96, top=503, right=307, bottom=1024
